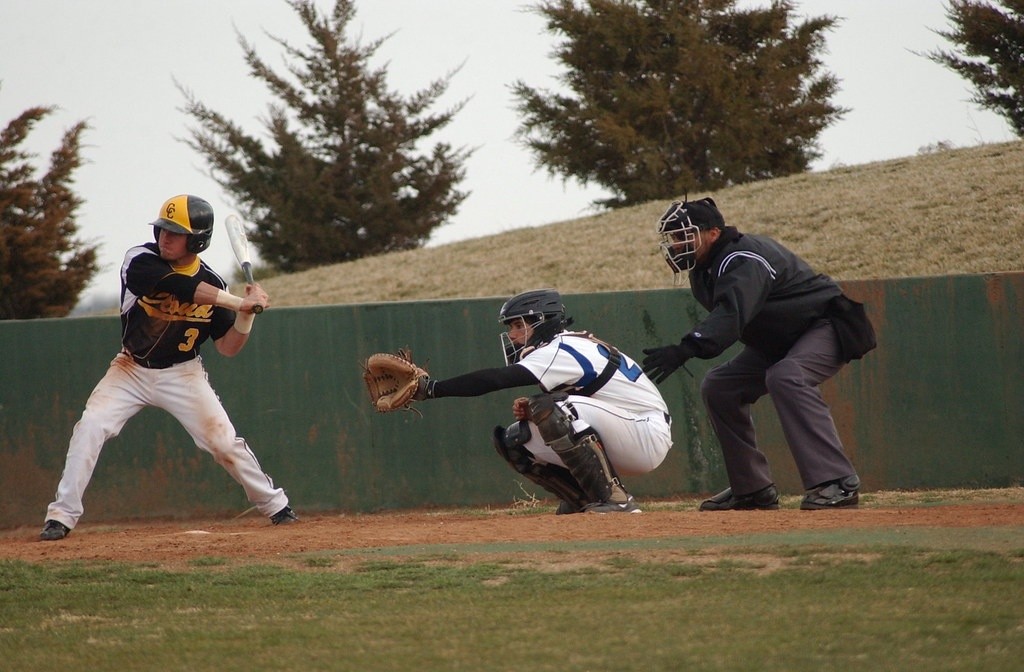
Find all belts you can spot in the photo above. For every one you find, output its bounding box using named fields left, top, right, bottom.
left=664, top=411, right=672, bottom=426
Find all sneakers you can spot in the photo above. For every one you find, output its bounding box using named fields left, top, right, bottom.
left=800, top=474, right=861, bottom=510
left=699, top=483, right=781, bottom=511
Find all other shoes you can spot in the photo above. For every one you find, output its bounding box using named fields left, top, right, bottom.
left=269, top=505, right=302, bottom=525
left=40, top=520, right=70, bottom=540
left=589, top=493, right=643, bottom=514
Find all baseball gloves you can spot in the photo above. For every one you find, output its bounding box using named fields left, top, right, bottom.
left=357, top=344, right=431, bottom=420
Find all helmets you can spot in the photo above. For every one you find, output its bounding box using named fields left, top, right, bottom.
left=656, top=197, right=725, bottom=286
left=151, top=196, right=214, bottom=254
left=498, top=288, right=575, bottom=367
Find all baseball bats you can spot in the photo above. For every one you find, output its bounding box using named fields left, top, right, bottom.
left=225, top=214, right=264, bottom=314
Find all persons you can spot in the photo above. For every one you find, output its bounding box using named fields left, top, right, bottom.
left=40, top=193, right=300, bottom=542
left=641, top=194, right=879, bottom=510
left=365, top=288, right=673, bottom=514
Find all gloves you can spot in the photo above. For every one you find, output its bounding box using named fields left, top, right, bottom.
left=642, top=344, right=689, bottom=384
